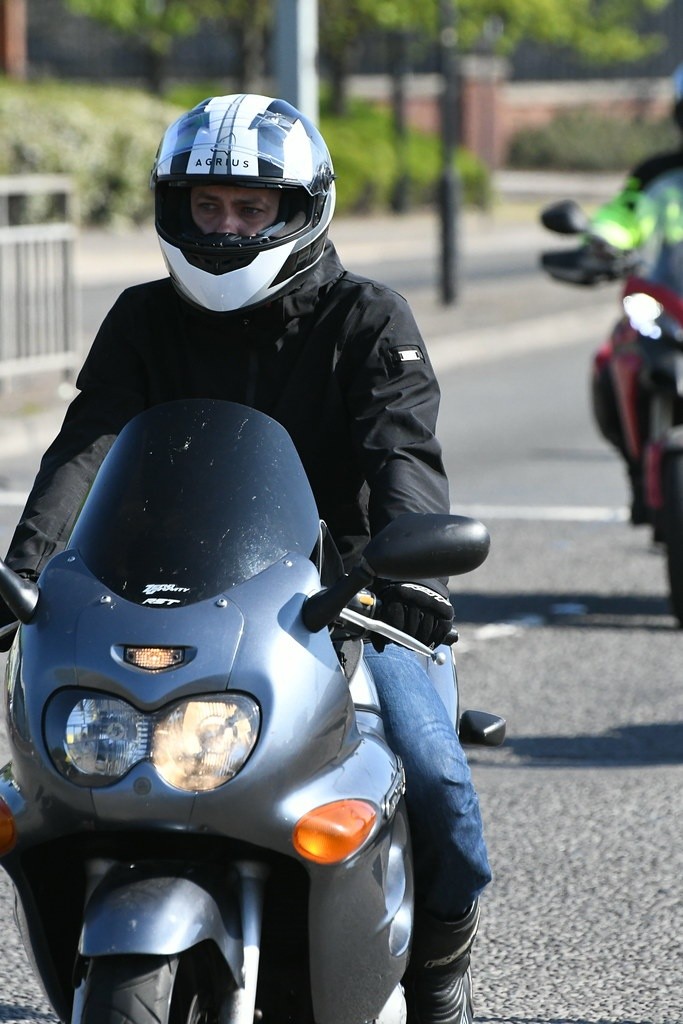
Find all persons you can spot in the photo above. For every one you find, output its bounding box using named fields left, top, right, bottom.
left=581, top=61, right=683, bottom=528
left=0, top=92, right=495, bottom=1024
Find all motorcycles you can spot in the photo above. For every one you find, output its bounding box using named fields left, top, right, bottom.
left=0, top=394, right=491, bottom=1024
left=540, top=201, right=683, bottom=629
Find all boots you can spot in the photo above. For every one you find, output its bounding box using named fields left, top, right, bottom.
left=400, top=895, right=479, bottom=1024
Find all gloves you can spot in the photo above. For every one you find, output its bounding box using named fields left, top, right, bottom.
left=0, top=574, right=37, bottom=652
left=369, top=582, right=454, bottom=653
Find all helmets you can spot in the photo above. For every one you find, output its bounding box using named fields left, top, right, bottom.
left=149, top=94, right=338, bottom=320
